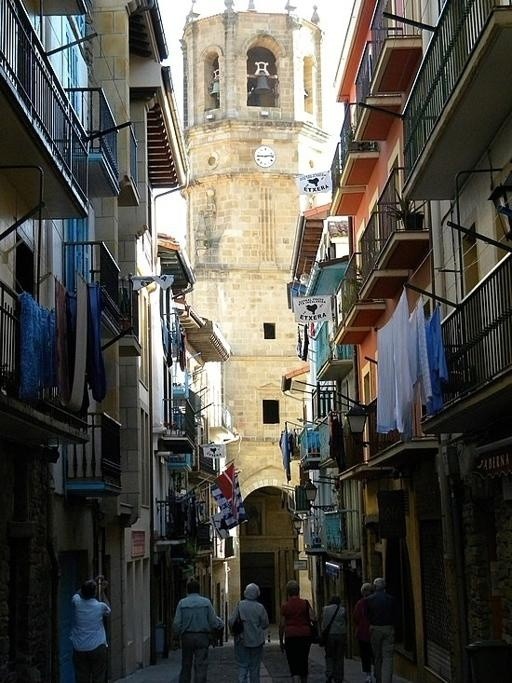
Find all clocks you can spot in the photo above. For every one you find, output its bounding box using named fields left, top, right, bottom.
left=253, top=144, right=277, bottom=169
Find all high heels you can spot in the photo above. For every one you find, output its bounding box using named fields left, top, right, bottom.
left=364, top=675, right=372, bottom=683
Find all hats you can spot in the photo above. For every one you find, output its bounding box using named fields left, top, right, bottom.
left=372, top=578, right=386, bottom=590
left=244, top=583, right=261, bottom=600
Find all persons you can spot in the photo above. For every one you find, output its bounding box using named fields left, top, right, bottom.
left=170, top=578, right=222, bottom=682
left=277, top=579, right=316, bottom=683
left=319, top=592, right=345, bottom=683
left=363, top=576, right=400, bottom=682
left=69, top=574, right=112, bottom=682
left=351, top=582, right=380, bottom=682
left=227, top=581, right=268, bottom=682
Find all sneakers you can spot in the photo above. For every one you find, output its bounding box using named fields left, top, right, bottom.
left=327, top=674, right=343, bottom=683
left=292, top=674, right=307, bottom=683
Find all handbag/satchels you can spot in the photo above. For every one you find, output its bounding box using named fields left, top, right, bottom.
left=352, top=624, right=359, bottom=639
left=320, top=625, right=333, bottom=647
left=310, top=626, right=320, bottom=644
left=231, top=619, right=244, bottom=635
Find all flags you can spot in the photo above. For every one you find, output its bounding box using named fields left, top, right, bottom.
left=210, top=459, right=249, bottom=540
